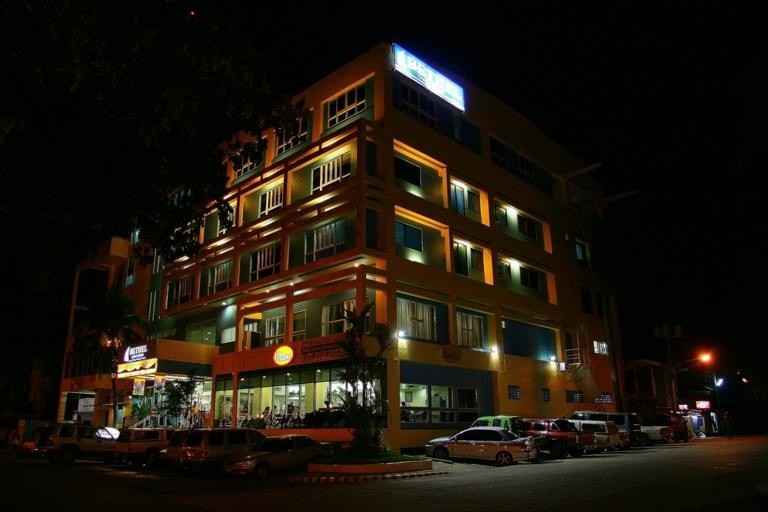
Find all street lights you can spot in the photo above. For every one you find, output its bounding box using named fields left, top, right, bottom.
left=669, top=349, right=716, bottom=412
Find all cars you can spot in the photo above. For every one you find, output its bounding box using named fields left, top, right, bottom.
left=261, top=434, right=335, bottom=472
left=418, top=407, right=689, bottom=468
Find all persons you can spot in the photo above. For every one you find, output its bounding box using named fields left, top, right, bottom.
left=400, top=401, right=410, bottom=413
left=191, top=399, right=298, bottom=420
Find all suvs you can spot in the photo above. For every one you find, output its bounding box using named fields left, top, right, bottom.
left=158, top=426, right=271, bottom=470
left=113, top=426, right=178, bottom=468
left=44, top=420, right=119, bottom=463
